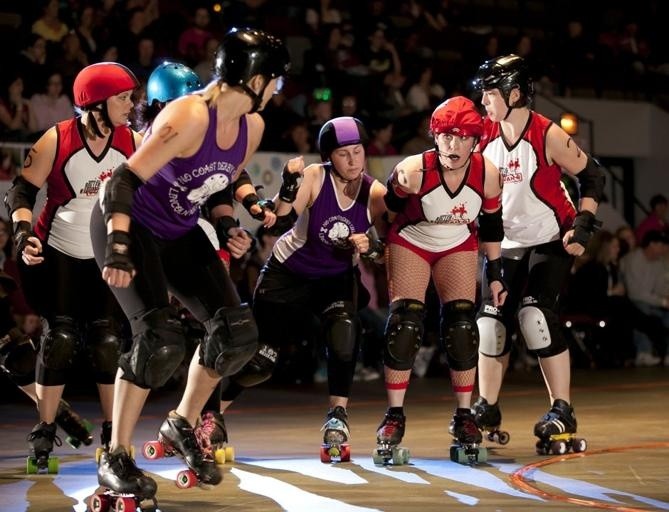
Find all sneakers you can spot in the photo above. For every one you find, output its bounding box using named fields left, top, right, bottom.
left=635, top=352, right=661, bottom=367
left=411, top=346, right=435, bottom=378
left=354, top=368, right=380, bottom=382
left=522, top=355, right=538, bottom=366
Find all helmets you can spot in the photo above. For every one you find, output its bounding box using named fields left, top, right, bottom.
left=146, top=62, right=204, bottom=107
left=472, top=53, right=532, bottom=108
left=430, top=95, right=484, bottom=137
left=319, top=117, right=369, bottom=162
left=214, top=29, right=292, bottom=87
left=73, top=62, right=140, bottom=107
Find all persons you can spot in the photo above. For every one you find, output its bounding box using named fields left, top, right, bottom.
left=374, top=96, right=508, bottom=466
left=1, top=0, right=669, bottom=157
left=471, top=52, right=606, bottom=458
left=0, top=29, right=293, bottom=512
left=562, top=194, right=669, bottom=365
left=205, top=116, right=388, bottom=463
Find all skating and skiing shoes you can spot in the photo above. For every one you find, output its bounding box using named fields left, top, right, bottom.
left=27, top=397, right=234, bottom=511
left=448, top=396, right=509, bottom=445
left=450, top=408, right=488, bottom=464
left=373, top=407, right=410, bottom=465
left=319, top=406, right=352, bottom=463
left=534, top=399, right=587, bottom=455
